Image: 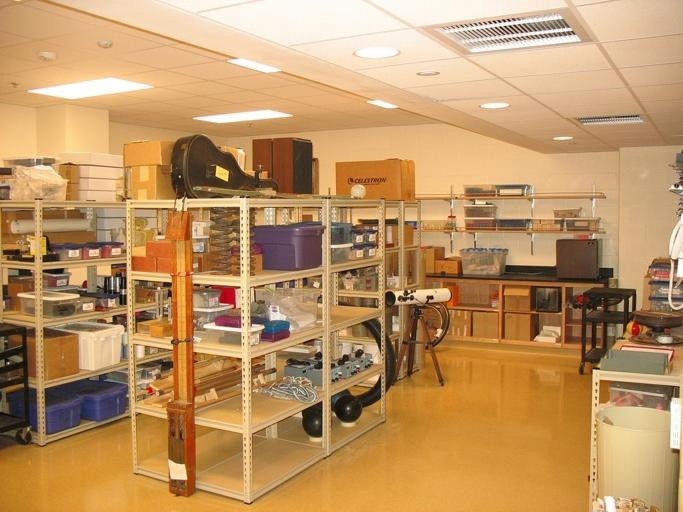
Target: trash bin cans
[595,406,679,512]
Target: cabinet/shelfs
[417,190,604,350]
[589,320,682,512]
[3,189,421,503]
[578,287,636,374]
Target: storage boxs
[251,137,312,194]
[124,140,177,199]
[335,158,415,202]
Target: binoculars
[385,288,452,314]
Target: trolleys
[0,323,31,444]
[579,287,636,374]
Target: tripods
[391,304,444,386]
